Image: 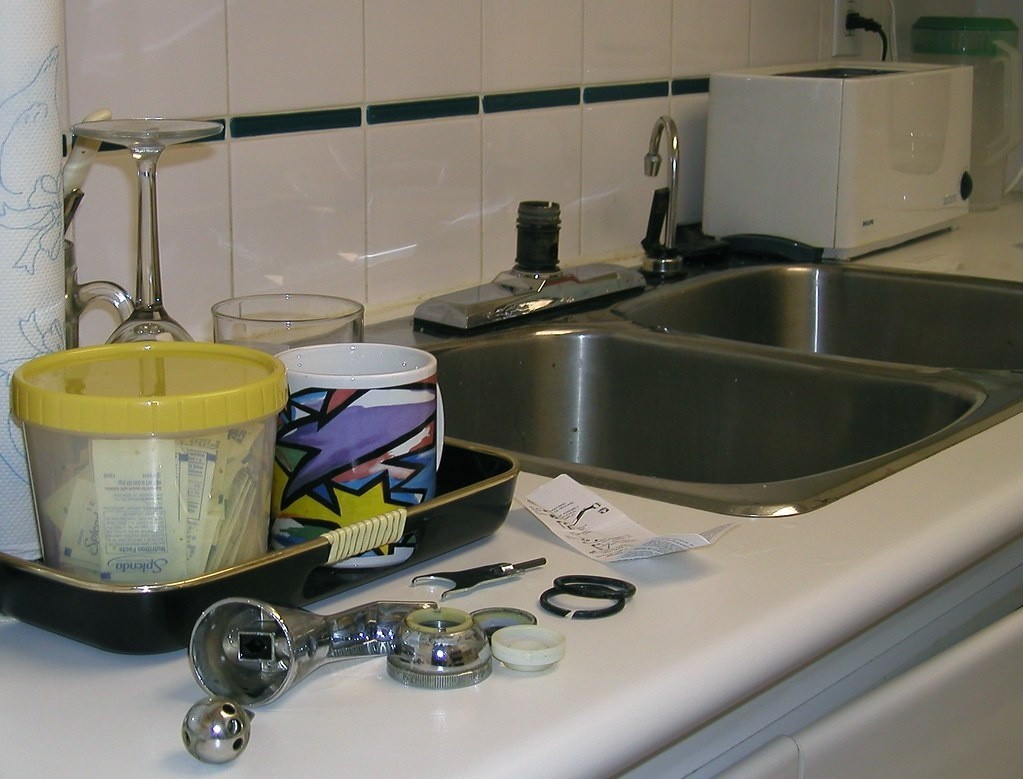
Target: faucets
[641,113,686,282]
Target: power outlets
[833,0,862,57]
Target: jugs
[911,13,1023,209]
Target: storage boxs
[11,341,289,587]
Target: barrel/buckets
[11,343,288,586]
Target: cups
[273,342,444,555]
[212,294,365,357]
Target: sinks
[614,261,1023,413]
[383,328,988,517]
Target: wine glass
[70,119,224,345]
